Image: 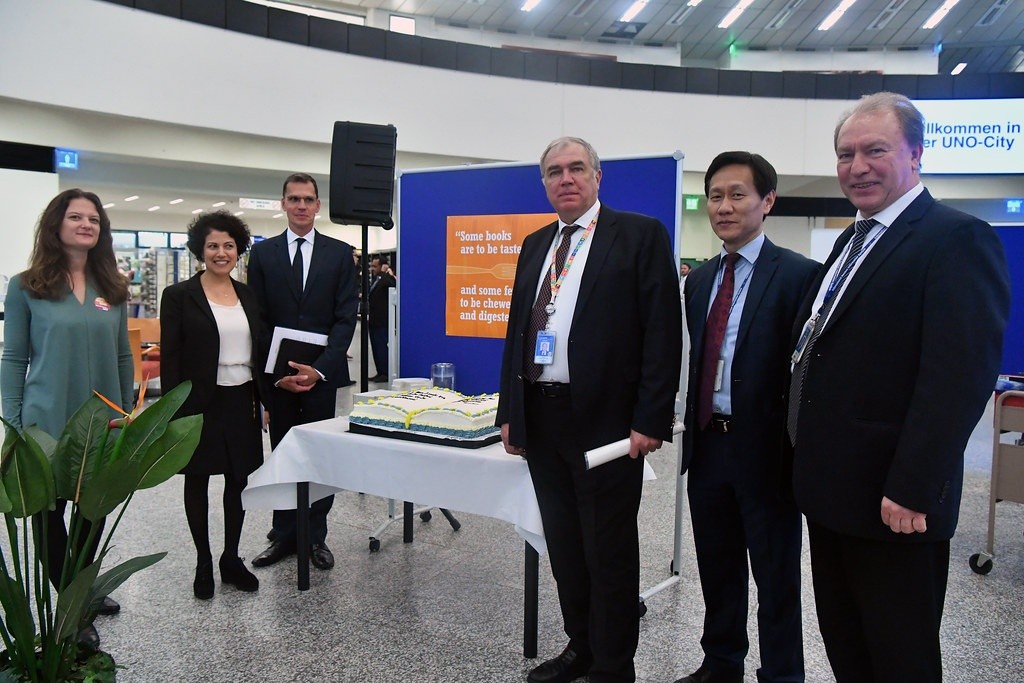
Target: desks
[241,415,684,675]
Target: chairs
[128,317,162,382]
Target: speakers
[330,120,397,230]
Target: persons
[247,173,358,571]
[680,262,691,294]
[336,245,397,388]
[495,136,682,683]
[790,91,1009,683]
[676,152,823,683]
[139,261,150,275]
[0,189,135,649]
[160,211,270,599]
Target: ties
[787,219,879,447]
[292,238,306,305]
[692,253,742,434]
[522,226,581,385]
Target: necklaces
[204,272,230,297]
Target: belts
[706,418,735,433]
[533,382,569,399]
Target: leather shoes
[251,540,298,568]
[527,640,591,683]
[78,623,101,650]
[311,542,335,570]
[97,594,120,614]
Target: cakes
[348,387,501,440]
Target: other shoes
[336,380,357,388]
[369,373,388,383]
[674,661,744,683]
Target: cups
[432,363,455,390]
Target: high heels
[193,551,259,599]
